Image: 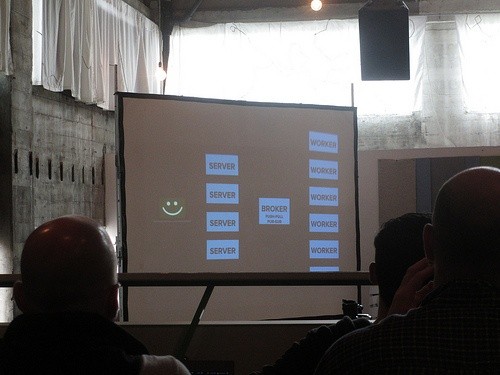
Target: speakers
[359,0,410,81]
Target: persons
[261,211,433,375]
[313,166,500,375]
[0,216,190,375]
